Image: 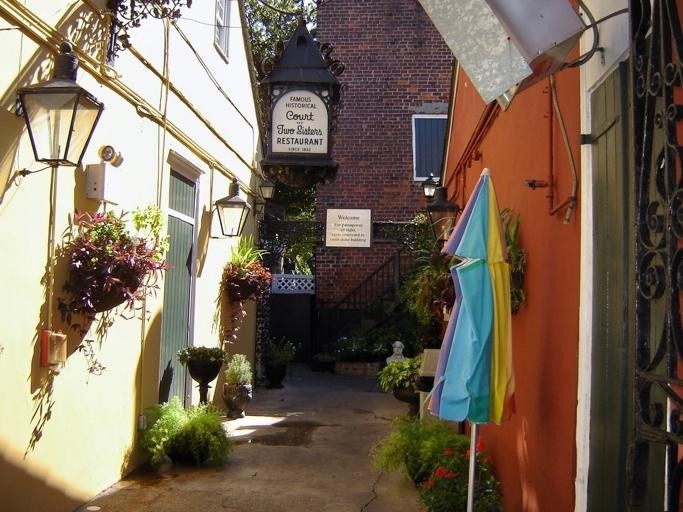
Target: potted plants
[59,204,170,373]
[222,233,273,346]
[177,344,228,403]
[140,395,234,470]
[223,353,253,418]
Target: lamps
[18,41,104,167]
[423,174,445,199]
[211,178,252,238]
[427,187,465,243]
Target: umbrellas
[427,168,527,511]
